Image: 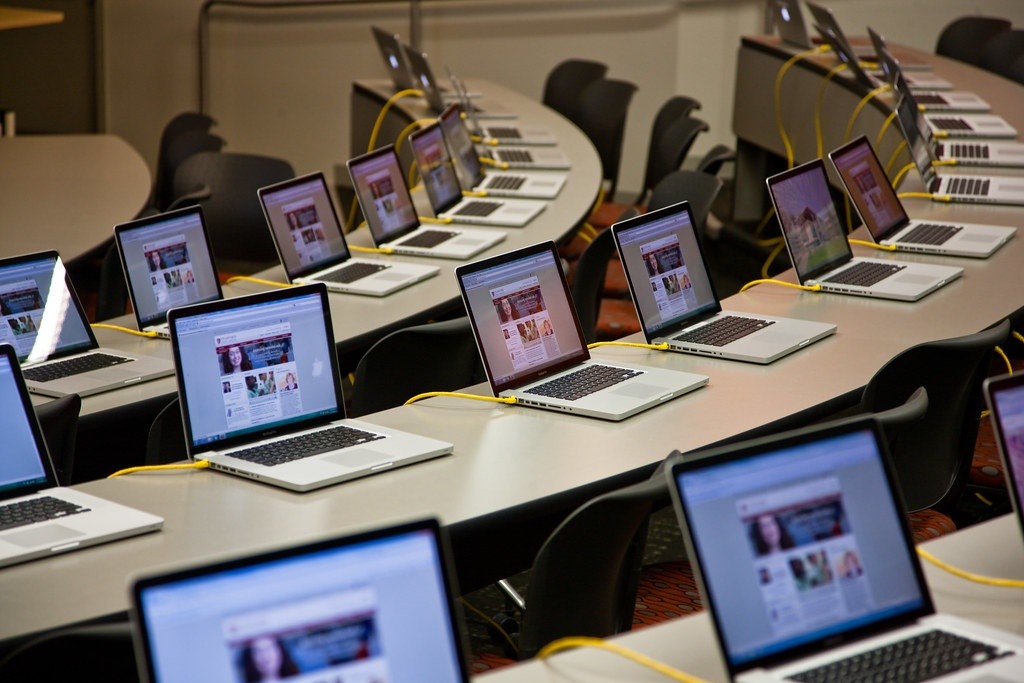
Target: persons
[149,251,195,286]
[645,253,693,293]
[371,183,381,199]
[497,296,554,340]
[286,211,323,245]
[222,346,298,392]
[234,634,296,681]
[746,512,863,585]
[7,314,37,333]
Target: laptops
[128,514,472,683]
[664,412,1024,683]
[0,0,1024,569]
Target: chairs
[0,16,1024,683]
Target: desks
[1,36,1024,644]
[26,81,602,430]
[0,135,153,265]
